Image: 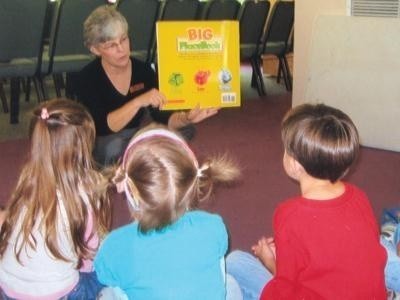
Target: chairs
[0,0,293,124]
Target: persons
[0,98,114,300]
[225,103,389,300]
[71,3,222,169]
[91,123,241,300]
